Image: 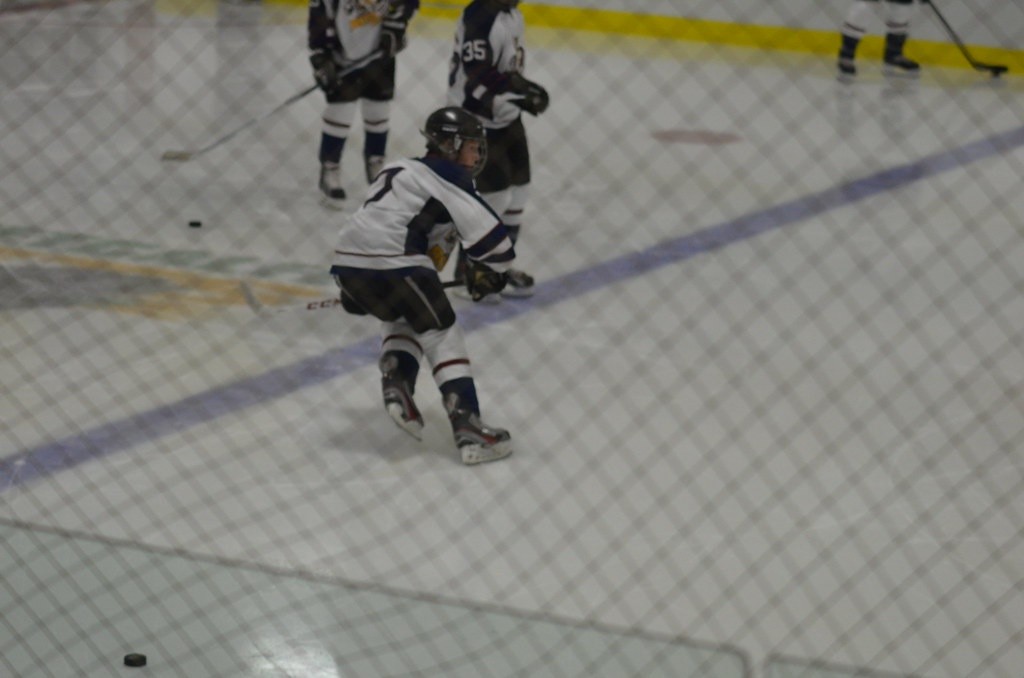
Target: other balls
[992,70,1000,77]
[123,653,146,667]
[188,220,202,228]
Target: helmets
[424,108,488,176]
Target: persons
[442,0,550,304]
[837,1,922,81]
[332,106,512,464]
[307,0,419,212]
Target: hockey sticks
[161,46,384,162]
[237,279,465,319]
[925,0,1009,72]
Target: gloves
[310,52,341,98]
[377,19,406,59]
[465,257,502,301]
[496,70,549,116]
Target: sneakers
[379,354,425,443]
[365,156,384,183]
[885,47,920,76]
[443,393,513,464]
[453,243,502,303]
[318,161,349,208]
[503,270,537,297]
[836,48,858,81]
